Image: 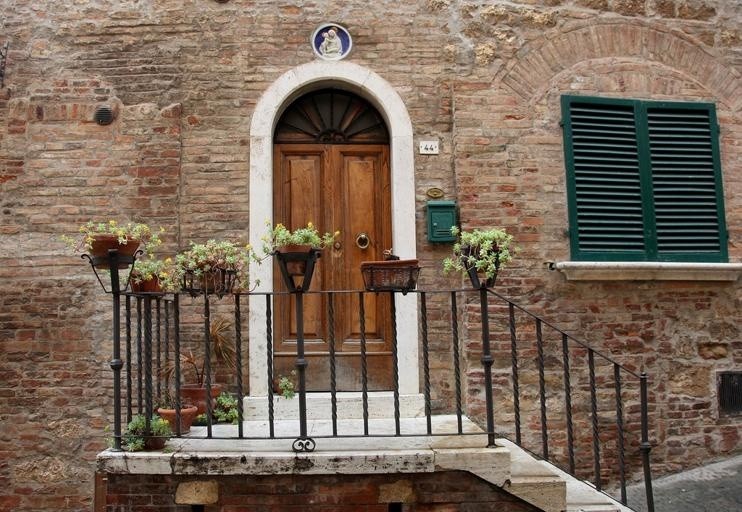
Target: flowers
[61,218,260,292]
[382,247,400,261]
[246,216,341,264]
[275,368,295,398]
[105,416,171,451]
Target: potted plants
[212,391,238,423]
[157,319,237,416]
[443,226,521,288]
[157,388,197,432]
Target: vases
[274,380,283,396]
[141,433,167,451]
[275,244,316,293]
[359,259,421,291]
